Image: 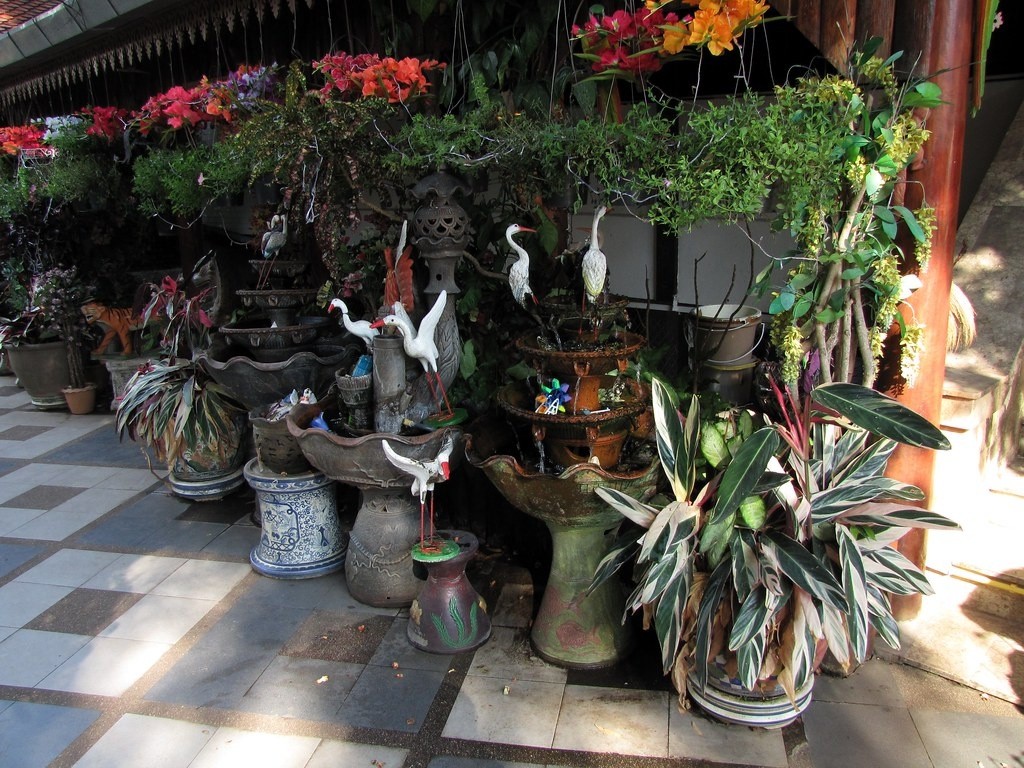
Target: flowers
[312,52,446,108]
[29,263,96,388]
[568,0,797,88]
[0,62,285,158]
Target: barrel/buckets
[690,304,766,365]
[693,356,762,407]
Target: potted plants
[586,370,963,729]
[0,310,96,410]
[113,274,247,502]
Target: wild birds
[328,288,458,423]
[506,223,539,310]
[575,202,615,308]
[254,214,291,289]
[381,435,456,554]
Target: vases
[61,383,96,414]
